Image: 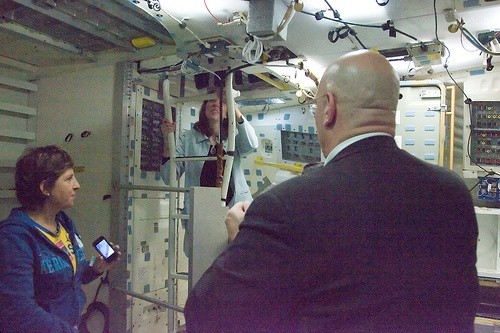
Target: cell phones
[92,236,118,263]
